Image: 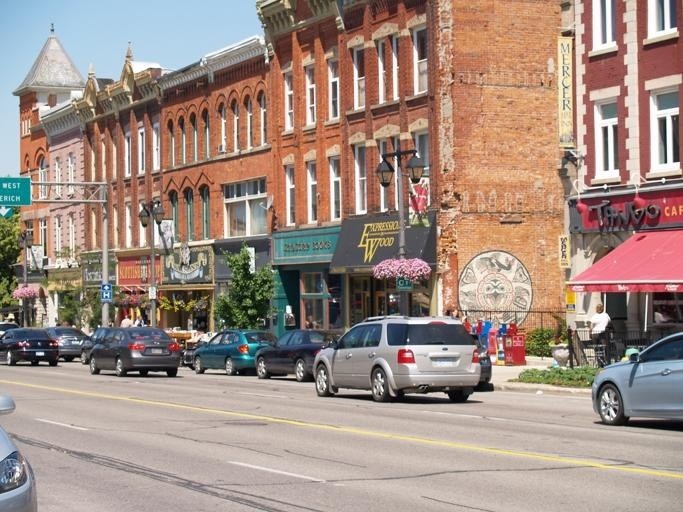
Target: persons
[590,304,611,367]
[120,314,148,328]
[63,319,77,328]
[654,305,668,324]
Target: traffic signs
[0,176,31,217]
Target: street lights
[375,147,425,317]
[18,231,34,328]
[138,200,165,326]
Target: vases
[550,344,569,367]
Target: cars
[0,396,37,511]
[592,333,683,425]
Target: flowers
[372,257,430,281]
[12,287,38,298]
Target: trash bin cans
[605,341,627,365]
